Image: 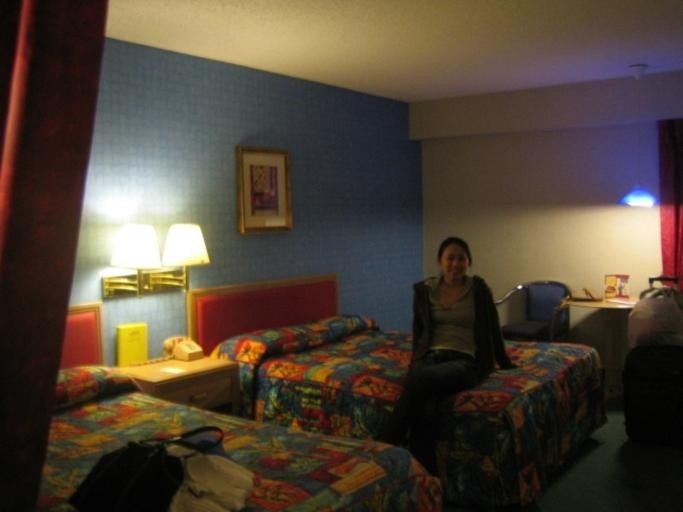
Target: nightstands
[115,356,243,417]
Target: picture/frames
[236,148,294,234]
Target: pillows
[53,366,142,411]
[220,313,377,367]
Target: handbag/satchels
[627,287,683,347]
[68,440,183,512]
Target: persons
[375,237,518,477]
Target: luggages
[622,277,683,443]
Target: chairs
[494,281,571,342]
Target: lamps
[100,223,212,302]
[617,64,659,208]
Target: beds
[187,274,605,506]
[40,302,443,512]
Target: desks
[560,291,640,408]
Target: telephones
[163,335,204,361]
